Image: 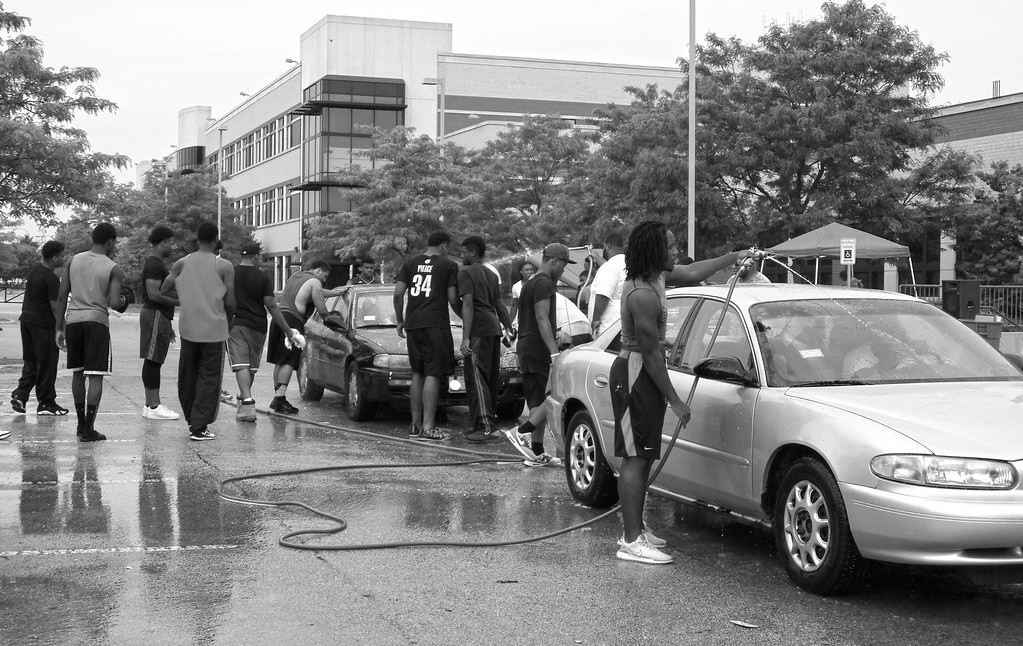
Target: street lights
[217,125,228,240]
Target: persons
[608,222,758,564]
[11,221,770,469]
[839,270,864,288]
[843,316,939,379]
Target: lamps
[286,59,298,66]
[240,92,251,98]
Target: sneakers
[523,452,562,468]
[37,399,69,415]
[616,520,668,548]
[505,425,538,461]
[616,530,674,563]
[142,403,179,420]
[10,394,26,413]
[188,425,216,440]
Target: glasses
[735,264,742,266]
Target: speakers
[941,280,980,321]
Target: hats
[239,242,261,255]
[542,242,578,265]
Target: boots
[80,404,107,442]
[75,402,86,436]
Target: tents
[761,222,919,299]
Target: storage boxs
[958,319,1003,352]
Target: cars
[542,281,1023,599]
[296,282,526,420]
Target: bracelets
[551,353,560,357]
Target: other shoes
[462,428,504,443]
[0,430,12,440]
[235,393,257,422]
[269,395,299,413]
[218,390,233,401]
[408,423,451,442]
